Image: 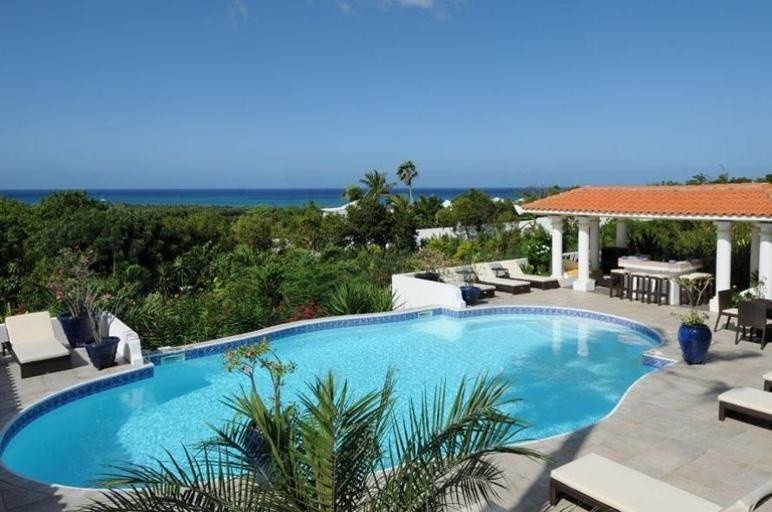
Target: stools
[630,270,650,305]
[608,269,631,300]
[647,274,671,307]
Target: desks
[740,282,771,314]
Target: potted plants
[80,276,140,370]
[673,275,714,370]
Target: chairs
[761,369,772,393]
[0,309,75,379]
[716,386,772,427]
[714,288,741,333]
[434,259,563,306]
[734,298,771,350]
[544,448,772,510]
